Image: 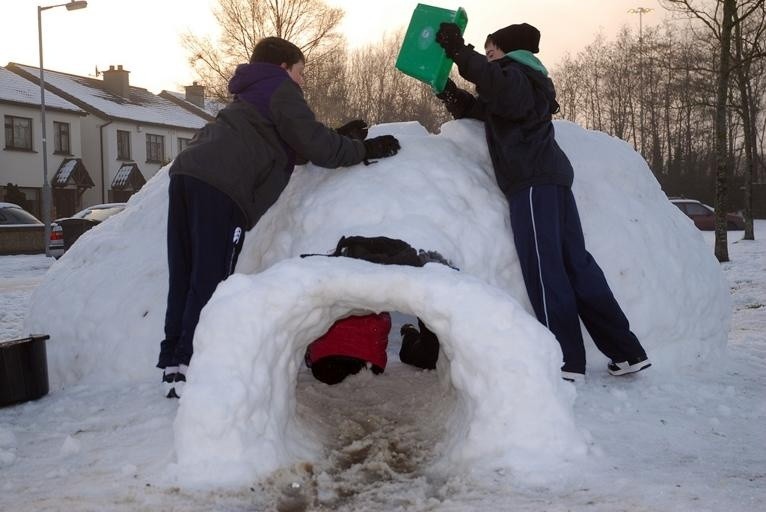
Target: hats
[492,23,540,54]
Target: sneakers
[607,355,652,376]
[161,372,186,398]
[561,370,585,382]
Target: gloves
[335,120,368,140]
[437,77,470,115]
[363,135,400,159]
[436,23,464,58]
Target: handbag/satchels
[343,236,422,267]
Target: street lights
[35,0,92,258]
[627,6,657,163]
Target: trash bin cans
[58,219,102,254]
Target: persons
[155,35,404,402]
[431,18,657,387]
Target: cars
[0,203,43,225]
[46,201,127,264]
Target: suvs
[667,193,745,231]
